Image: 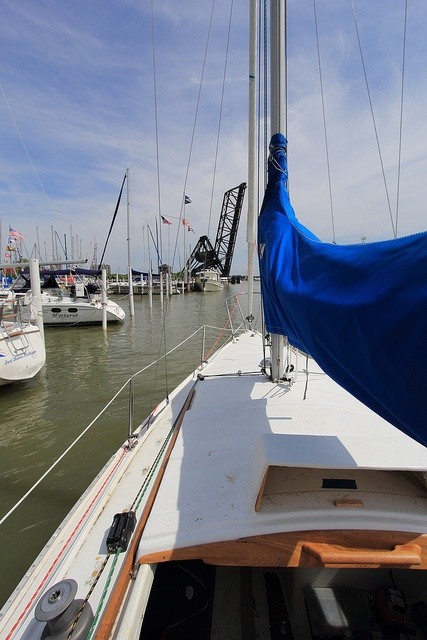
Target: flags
[185,194,192,205]
[8,226,25,239]
[180,218,196,235]
[161,215,172,225]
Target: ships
[195,269,224,292]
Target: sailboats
[0,225,103,313]
[109,167,181,294]
[0,0,427,640]
[16,233,126,323]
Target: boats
[0,299,46,386]
[221,277,228,284]
